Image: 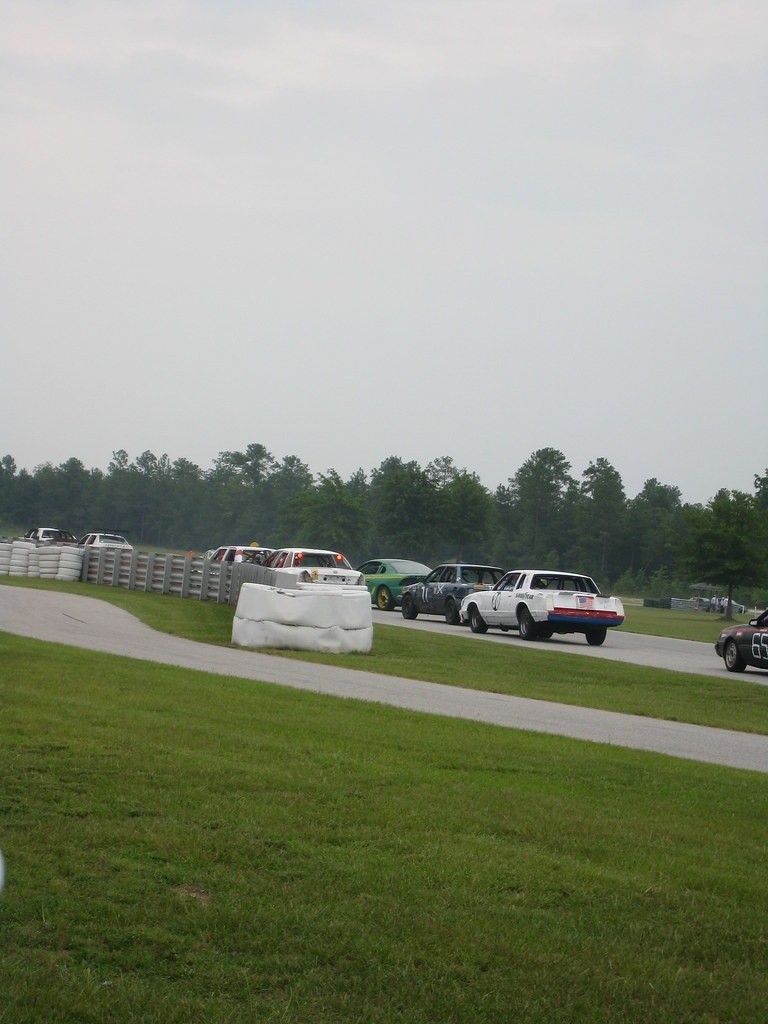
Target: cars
[725,598,747,614]
[400,563,515,626]
[191,545,276,575]
[77,532,134,552]
[715,607,768,672]
[353,558,439,612]
[458,569,625,646]
[262,547,366,587]
[17,527,78,542]
